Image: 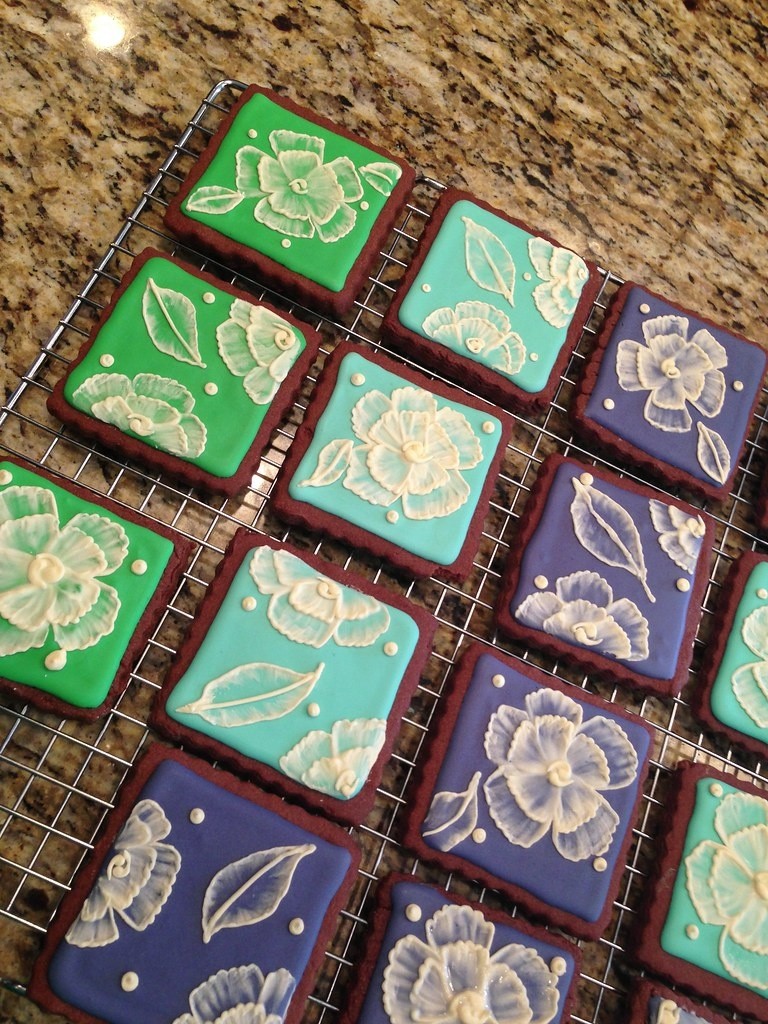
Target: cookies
[0,79,768,1024]
